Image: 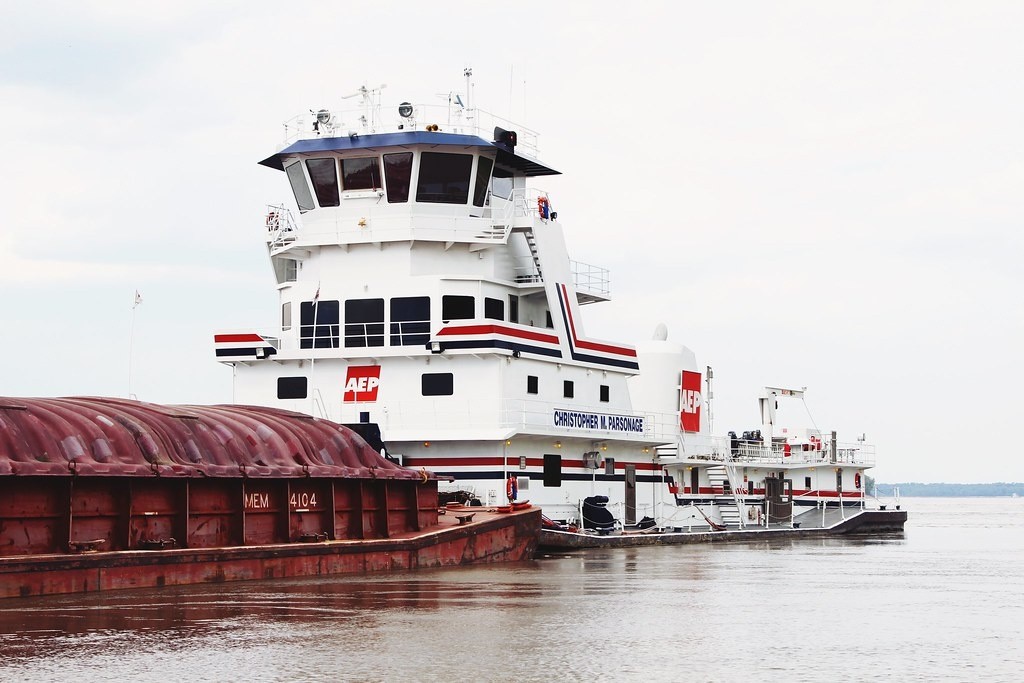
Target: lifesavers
[507,477,518,500]
[855,473,861,488]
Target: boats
[214,67,907,547]
